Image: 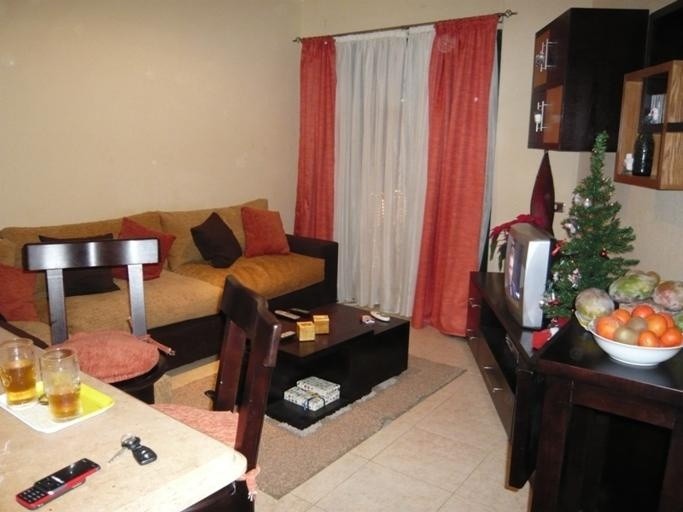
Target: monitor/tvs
[503,222,567,330]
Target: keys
[109,433,157,465]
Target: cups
[0,338,39,410]
[40,347,81,423]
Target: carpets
[159,339,467,499]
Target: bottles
[631,124,655,178]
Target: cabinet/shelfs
[527,88,621,152]
[532,7,647,87]
[614,60,683,193]
[460,272,562,493]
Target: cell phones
[16,459,100,510]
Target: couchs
[1,199,339,372]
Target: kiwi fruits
[627,316,647,331]
[614,325,639,346]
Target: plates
[0,381,115,434]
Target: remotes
[290,307,309,314]
[274,310,301,321]
[371,310,390,321]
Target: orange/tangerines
[660,325,683,347]
[610,308,632,326]
[631,304,655,319]
[657,311,674,328]
[644,314,667,337]
[596,315,622,340]
[637,330,659,348]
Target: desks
[1,330,248,512]
[532,295,683,512]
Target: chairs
[23,236,164,423]
[142,275,282,511]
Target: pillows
[37,233,120,300]
[113,217,177,281]
[189,212,242,268]
[0,262,37,323]
[241,206,291,259]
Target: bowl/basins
[588,320,683,366]
